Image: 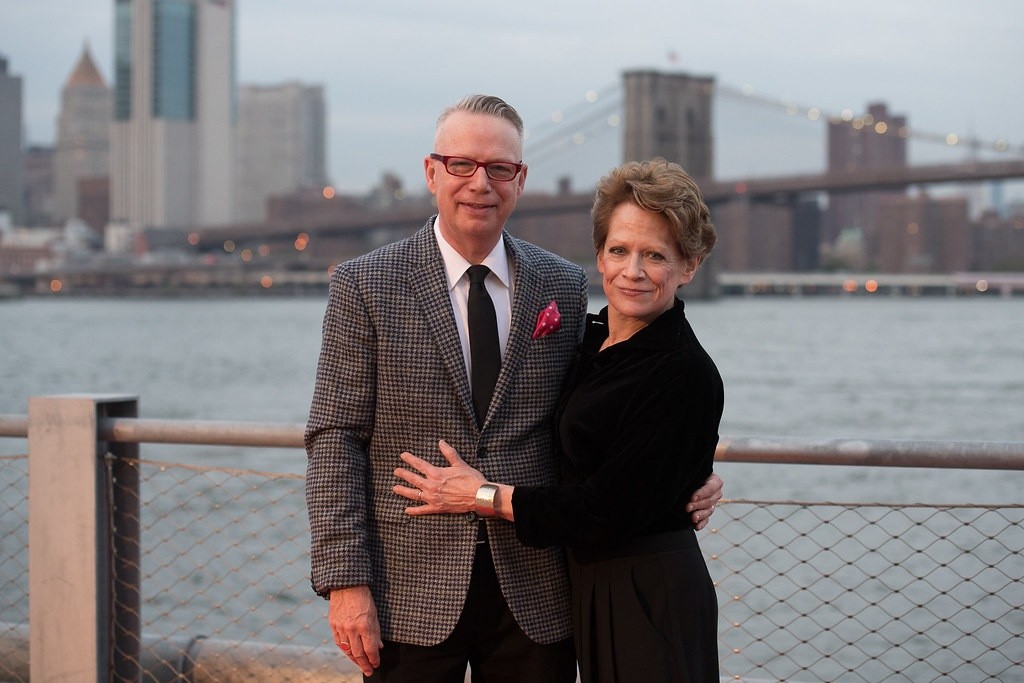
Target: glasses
[428,150,520,182]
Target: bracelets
[475,485,498,517]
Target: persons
[299,93,588,682]
[392,160,725,682]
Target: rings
[418,490,422,501]
[711,505,715,512]
[340,642,350,651]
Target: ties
[465,265,501,430]
